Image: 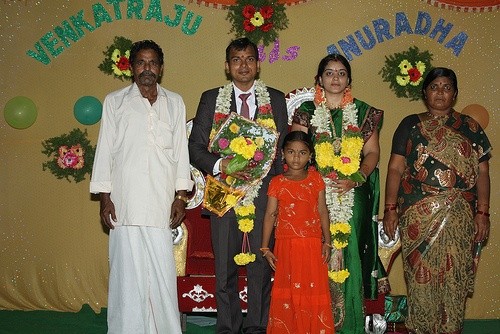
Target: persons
[262,131,335,334]
[189,38,288,334]
[383,67,494,334]
[90,40,187,334]
[292,54,391,334]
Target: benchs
[171,118,403,334]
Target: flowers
[212,117,277,205]
[41,128,97,183]
[377,45,435,101]
[96,35,134,83]
[224,0,289,47]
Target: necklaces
[209,79,277,265]
[311,84,364,248]
[427,112,445,118]
[325,98,345,153]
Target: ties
[239,93,250,120]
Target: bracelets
[263,250,270,257]
[477,211,491,217]
[355,170,367,188]
[384,207,396,214]
[476,204,490,208]
[385,204,397,206]
[324,241,334,249]
[174,195,187,203]
[260,248,270,252]
